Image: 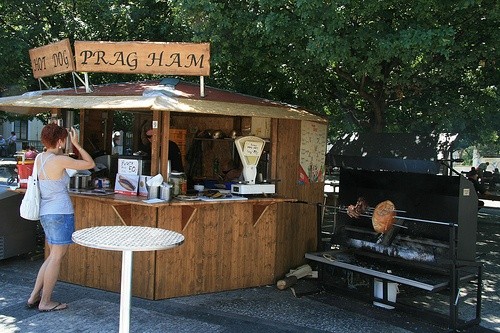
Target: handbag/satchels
[20,153,42,221]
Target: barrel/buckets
[16,164,33,190]
[373,277,399,310]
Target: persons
[6,131,17,143]
[0,135,6,144]
[25,145,47,159]
[23,123,95,313]
[143,124,183,177]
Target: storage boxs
[169,129,187,169]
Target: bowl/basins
[159,184,174,201]
[146,185,160,200]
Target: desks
[71,225,184,333]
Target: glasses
[145,134,152,139]
[58,126,62,130]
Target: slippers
[38,302,68,312]
[25,296,41,308]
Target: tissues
[146,173,163,199]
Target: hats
[0,135,4,138]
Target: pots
[70,174,93,190]
